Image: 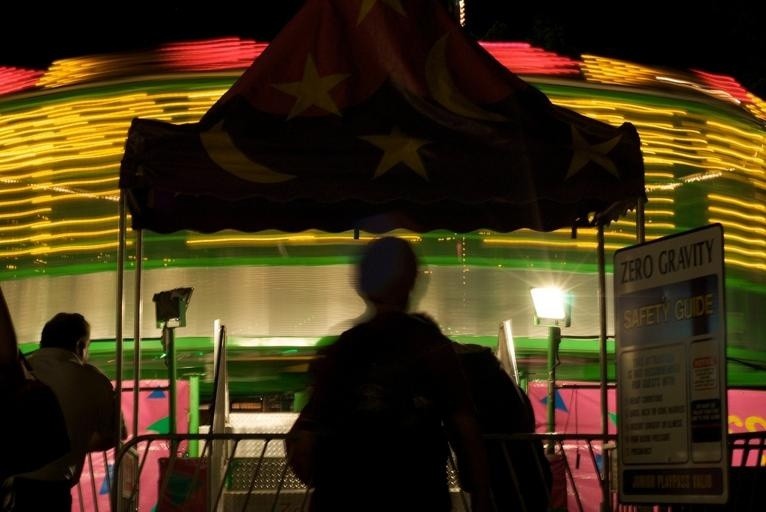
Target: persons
[0,313,127,512]
[0,289,71,512]
[306,237,490,512]
[452,345,552,512]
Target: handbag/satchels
[17,350,72,475]
